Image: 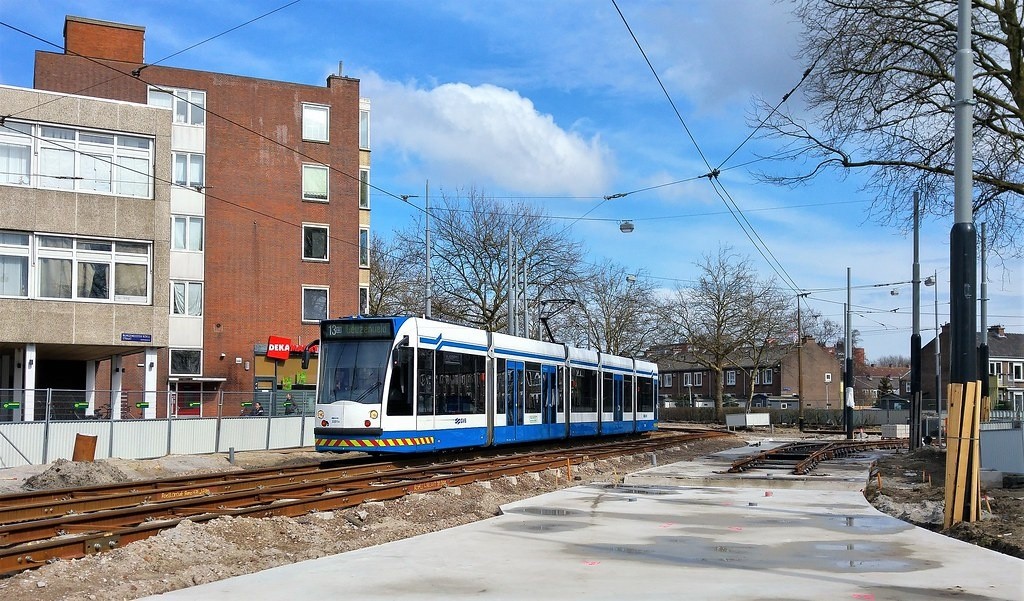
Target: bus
[300,314,661,458]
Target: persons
[247,401,264,416]
[281,392,298,415]
